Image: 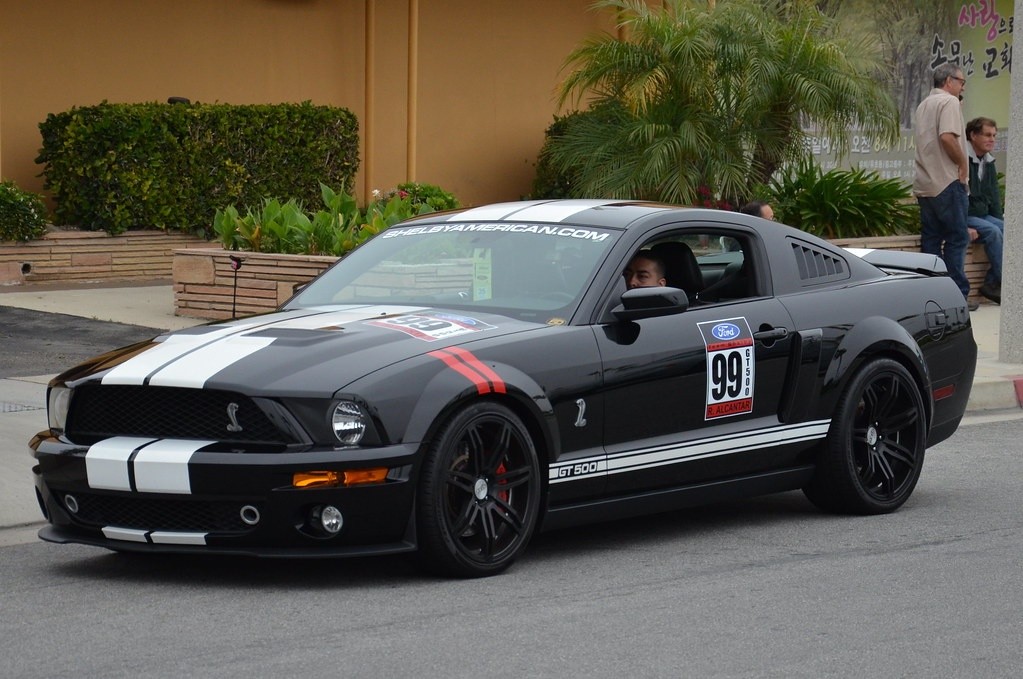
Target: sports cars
[29,198,980,579]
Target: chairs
[651,241,718,308]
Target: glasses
[951,76,966,86]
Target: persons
[623,250,669,292]
[955,118,1007,308]
[727,203,782,252]
[913,63,980,312]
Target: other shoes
[979,285,1001,304]
[966,301,979,311]
